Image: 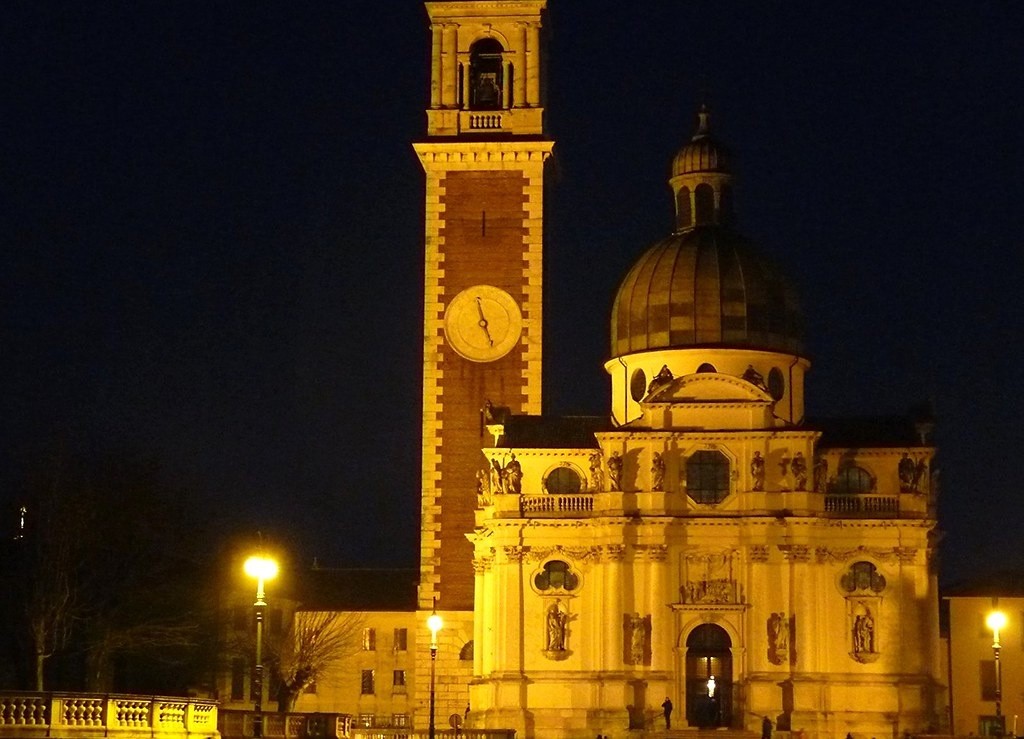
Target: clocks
[443,285,523,363]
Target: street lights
[242,544,279,738]
[983,610,1007,738]
[426,595,444,738]
